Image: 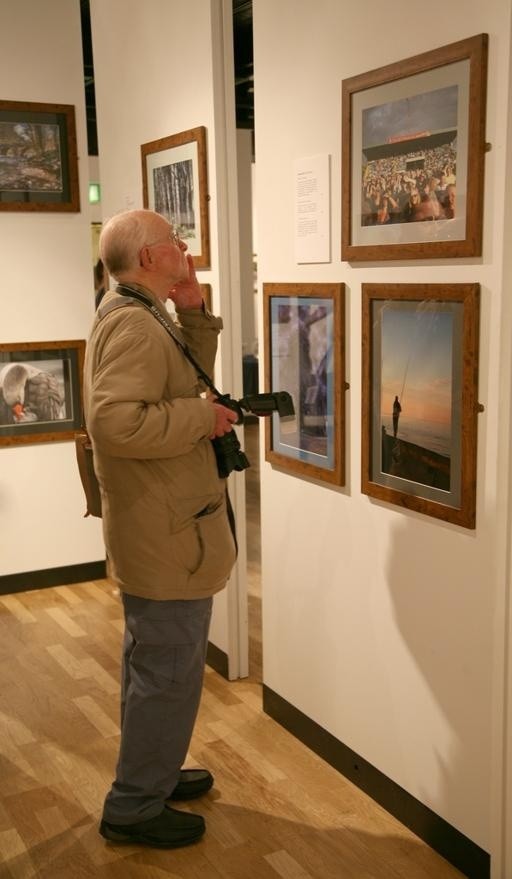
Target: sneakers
[166,768,215,802]
[97,803,208,849]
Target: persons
[392,395,401,437]
[361,142,456,226]
[12,401,37,423]
[84,209,237,851]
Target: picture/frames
[0,338,87,449]
[0,97,82,214]
[261,279,347,486]
[359,282,480,533]
[341,32,489,259]
[141,124,211,270]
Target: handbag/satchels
[73,295,144,519]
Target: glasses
[410,194,417,198]
[148,229,180,247]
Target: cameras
[210,390,297,479]
